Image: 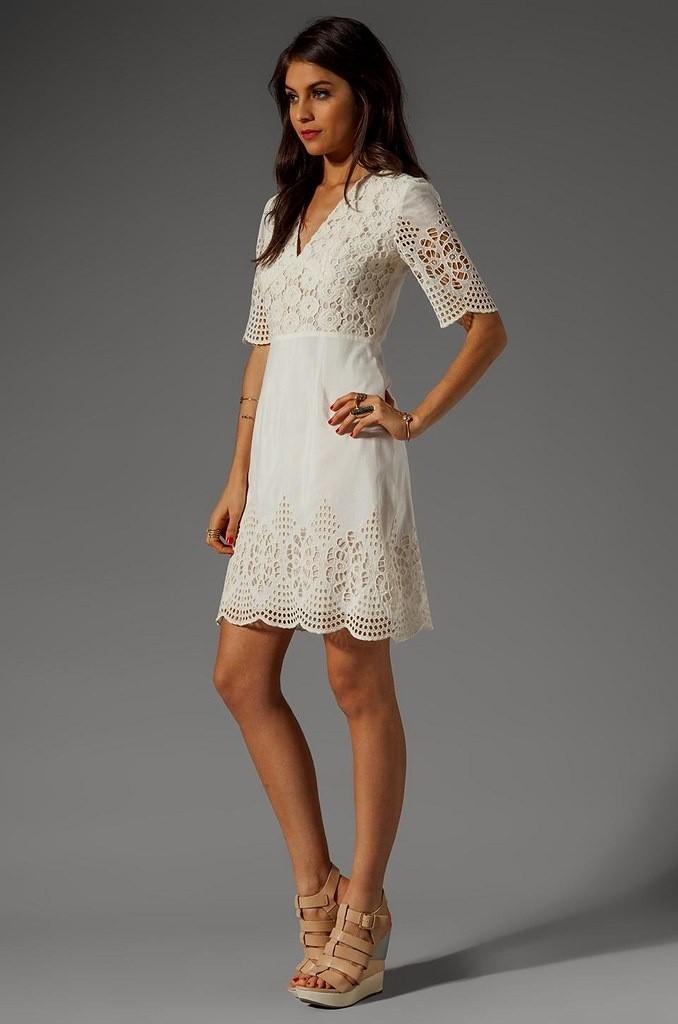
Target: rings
[206,528,221,539]
[351,390,374,415]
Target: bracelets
[239,393,258,420]
[402,411,412,443]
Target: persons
[201,17,509,1008]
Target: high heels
[297,889,392,1008]
[287,862,340,999]
